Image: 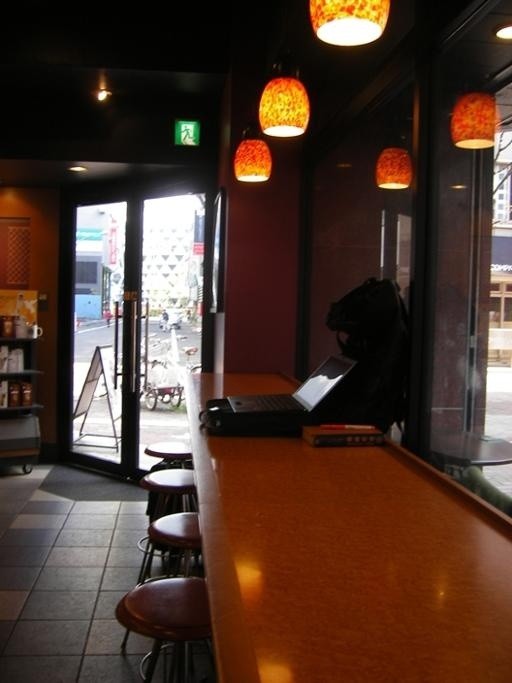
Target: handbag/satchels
[198,398,303,438]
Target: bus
[165,296,197,320]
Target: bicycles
[152,336,202,376]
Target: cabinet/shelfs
[2,333,43,466]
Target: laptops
[226,351,358,414]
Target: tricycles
[140,360,184,410]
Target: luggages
[145,457,199,555]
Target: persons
[161,309,169,332]
[105,309,113,325]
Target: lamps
[450,87,502,150]
[310,1,391,48]
[375,144,413,191]
[259,52,310,139]
[233,119,272,187]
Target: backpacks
[325,277,414,434]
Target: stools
[116,441,213,683]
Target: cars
[159,312,182,329]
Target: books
[304,423,385,447]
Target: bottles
[0,315,44,410]
